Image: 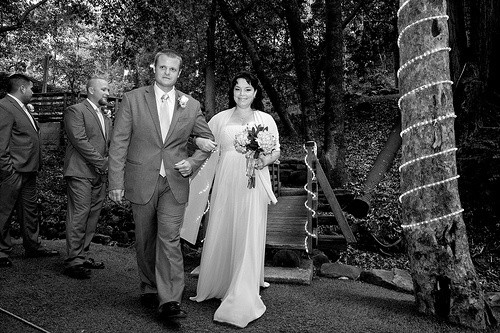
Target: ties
[23,106,35,128]
[159,94,169,178]
[95,108,105,137]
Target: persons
[107,48,216,320]
[179,70,281,328]
[63,78,112,276]
[0,74,60,268]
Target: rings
[257,164,259,168]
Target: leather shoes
[0,257,12,268]
[82,259,104,268]
[29,249,58,257]
[158,301,187,317]
[64,265,91,275]
[140,291,158,309]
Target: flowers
[104,109,111,118]
[26,103,35,112]
[177,95,189,108]
[233,122,281,188]
